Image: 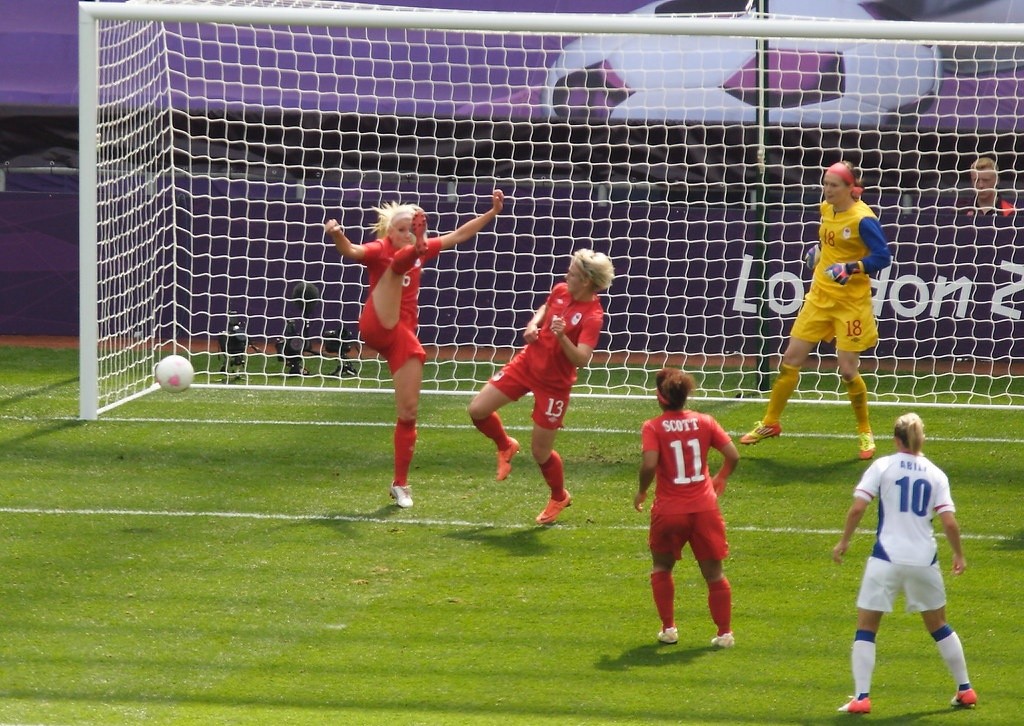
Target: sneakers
[838,696,871,713]
[496,437,520,480]
[951,689,976,707]
[858,431,875,460]
[711,633,734,647]
[389,480,413,507]
[658,627,678,643]
[535,489,571,524]
[411,211,429,255]
[742,423,781,445]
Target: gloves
[826,262,853,285]
[803,244,820,270]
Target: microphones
[292,282,320,310]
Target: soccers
[157,354,196,391]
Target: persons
[324,189,504,508]
[635,368,739,647]
[467,249,615,525]
[833,413,978,713]
[962,157,1022,216]
[739,160,891,460]
